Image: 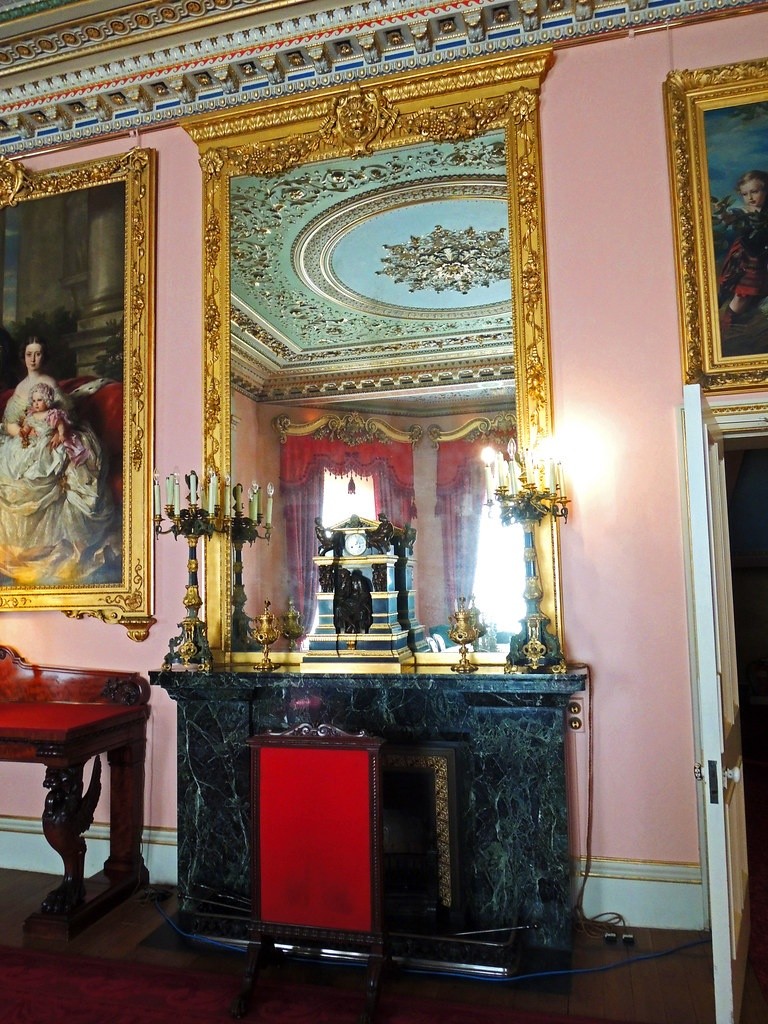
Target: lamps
[151,465,231,671]
[230,481,274,652]
[482,437,571,675]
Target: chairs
[432,633,446,651]
[425,636,438,653]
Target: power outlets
[566,698,584,732]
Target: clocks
[303,513,411,663]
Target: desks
[0,645,150,943]
[441,643,511,653]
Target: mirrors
[181,46,564,668]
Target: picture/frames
[0,146,157,644]
[662,55,768,396]
